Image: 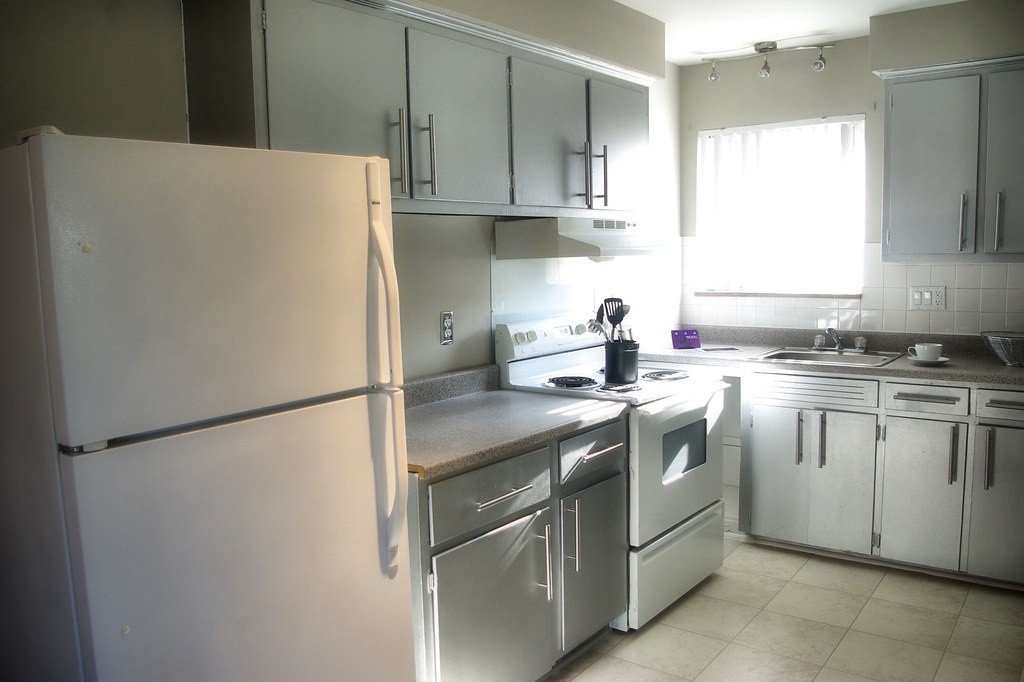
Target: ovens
[603,380,723,633]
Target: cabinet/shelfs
[738,370,880,566]
[408,417,628,682]
[880,56,1024,263]
[877,376,1024,591]
[183,1,652,216]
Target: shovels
[604,298,624,342]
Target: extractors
[490,216,659,263]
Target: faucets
[826,326,843,349]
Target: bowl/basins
[980,329,1024,365]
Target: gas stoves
[494,314,724,406]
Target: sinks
[762,346,895,366]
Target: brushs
[586,319,613,343]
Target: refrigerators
[0,124,418,682]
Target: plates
[908,356,950,366]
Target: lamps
[708,61,720,82]
[812,47,825,72]
[757,53,771,79]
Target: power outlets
[909,286,945,310]
[439,311,454,345]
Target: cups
[603,340,639,384]
[908,343,943,360]
